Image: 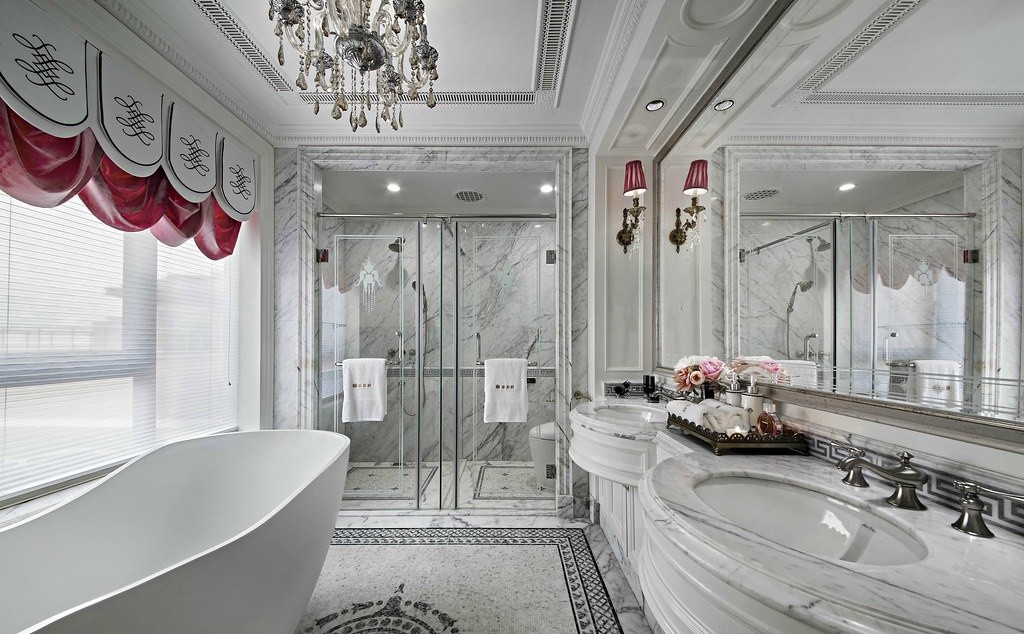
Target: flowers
[732,355,790,386]
[672,353,736,402]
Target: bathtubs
[0,427,352,634]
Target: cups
[725,389,744,407]
[741,392,765,427]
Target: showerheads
[787,280,814,312]
[805,233,833,253]
[411,280,428,310]
[388,236,406,253]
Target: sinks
[691,469,929,565]
[593,403,670,423]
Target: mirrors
[651,0,1024,458]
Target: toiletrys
[724,372,783,438]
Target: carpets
[294,526,625,634]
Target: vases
[700,381,716,400]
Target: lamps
[267,0,440,134]
[614,159,647,255]
[669,159,709,254]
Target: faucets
[651,390,686,406]
[836,450,930,512]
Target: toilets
[528,419,571,491]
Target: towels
[905,359,965,414]
[483,358,529,424]
[765,359,819,391]
[342,358,389,424]
[666,398,751,437]
